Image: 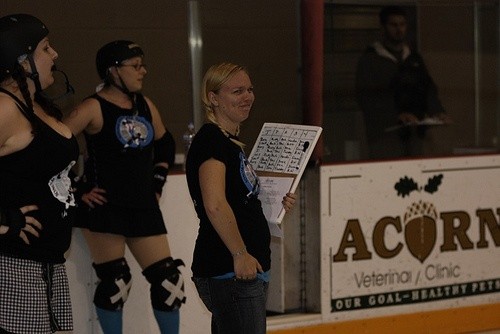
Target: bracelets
[231,246,246,257]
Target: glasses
[120,63,148,71]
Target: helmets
[0,13,49,63]
[96,40,144,80]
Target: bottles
[183,121,195,171]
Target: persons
[63,39,186,334]
[185,64,298,334]
[0,14,80,334]
[359,5,447,157]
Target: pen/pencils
[296,141,309,170]
